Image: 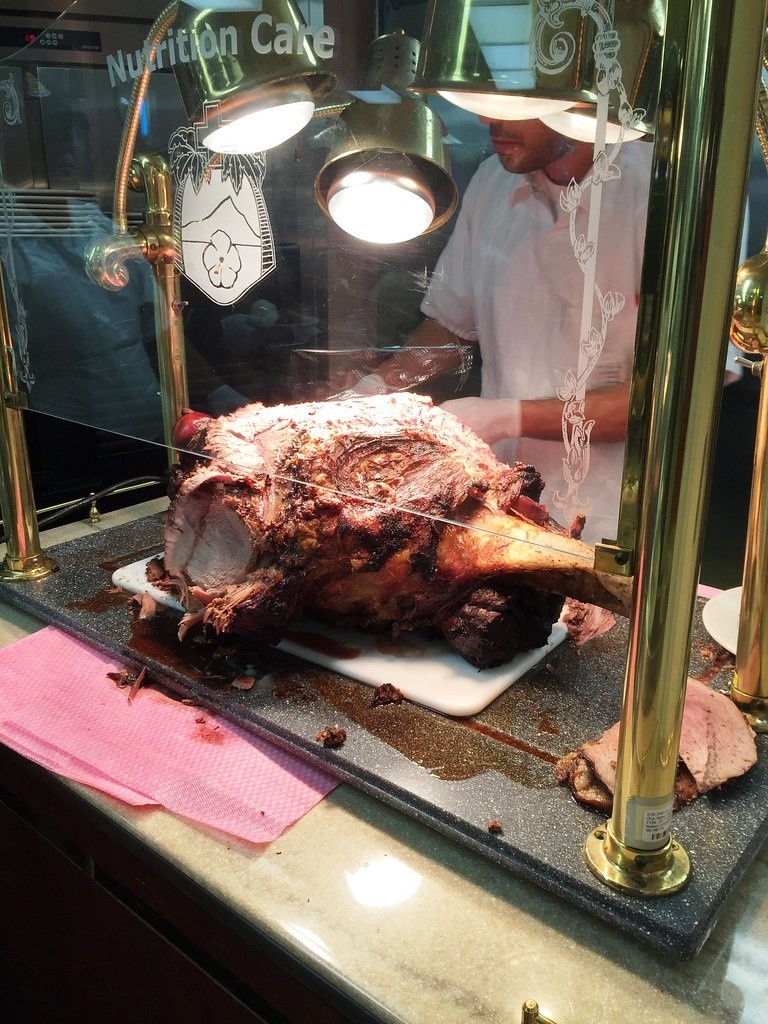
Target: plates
[702,586,743,655]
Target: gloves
[436,397,522,445]
[351,373,388,395]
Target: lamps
[203,27,458,245]
[405,0,768,178]
[82,0,338,292]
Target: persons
[0,86,253,538]
[334,114,751,552]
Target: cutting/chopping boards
[110,550,568,717]
